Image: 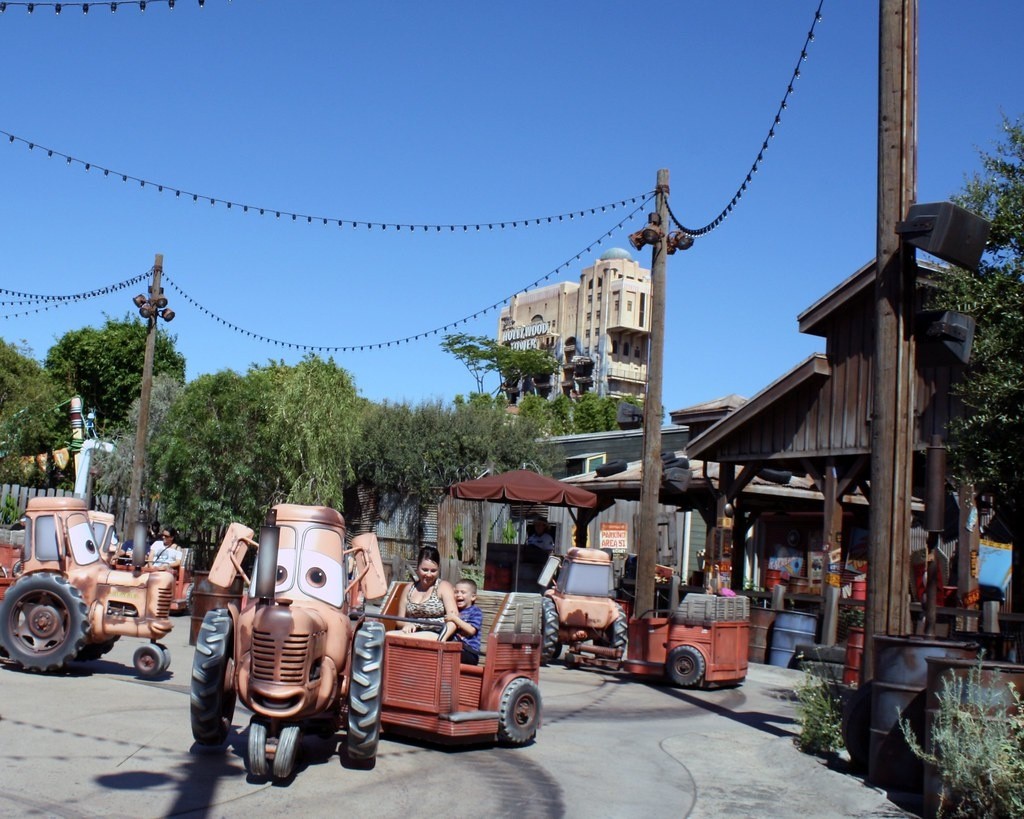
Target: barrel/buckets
[768,610,817,668]
[850,579,866,617]
[692,569,703,586]
[788,575,808,594]
[748,605,777,665]
[765,568,781,592]
[189,571,244,646]
[923,656,1024,819]
[864,633,979,795]
[840,625,864,774]
[617,598,631,626]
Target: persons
[386,546,460,642]
[111,526,153,568]
[527,516,554,555]
[444,578,483,665]
[145,527,182,575]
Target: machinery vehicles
[188,504,546,780]
[0,497,194,678]
[537,546,752,688]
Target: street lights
[625,168,694,619]
[127,253,175,530]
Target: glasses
[161,534,171,538]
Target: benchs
[366,581,542,711]
[642,592,750,663]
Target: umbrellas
[451,462,598,591]
